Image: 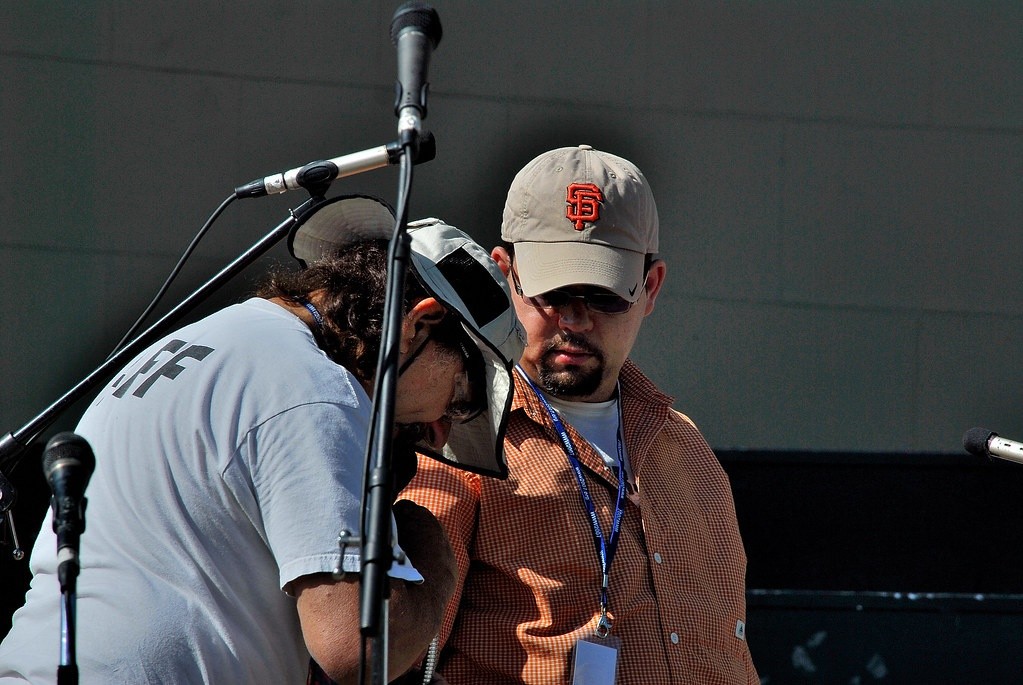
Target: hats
[286,194,528,482]
[501,144,659,303]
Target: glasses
[510,258,650,316]
[442,318,487,424]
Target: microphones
[963,427,1023,464]
[43,432,95,593]
[235,130,437,198]
[390,0,442,147]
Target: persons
[1,215,526,685]
[397,143,767,685]
